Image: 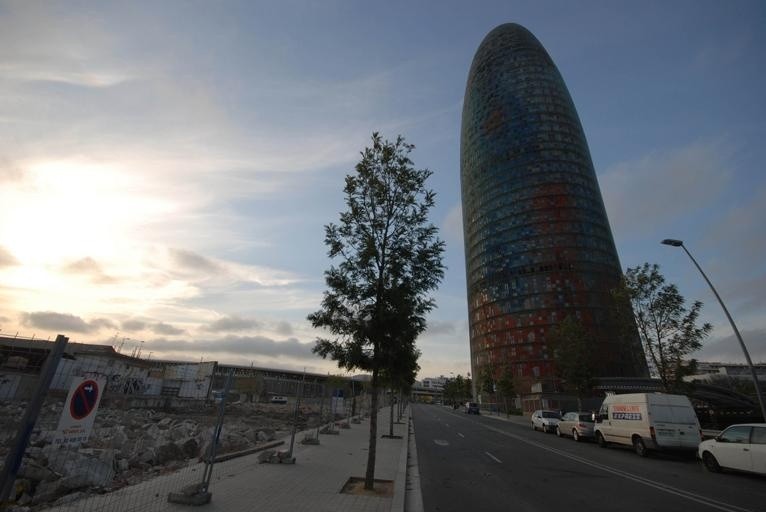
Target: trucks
[593,391,704,459]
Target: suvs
[465,402,480,414]
[532,409,562,432]
[556,411,595,441]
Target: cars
[697,423,766,477]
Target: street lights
[659,237,766,420]
[450,371,456,383]
